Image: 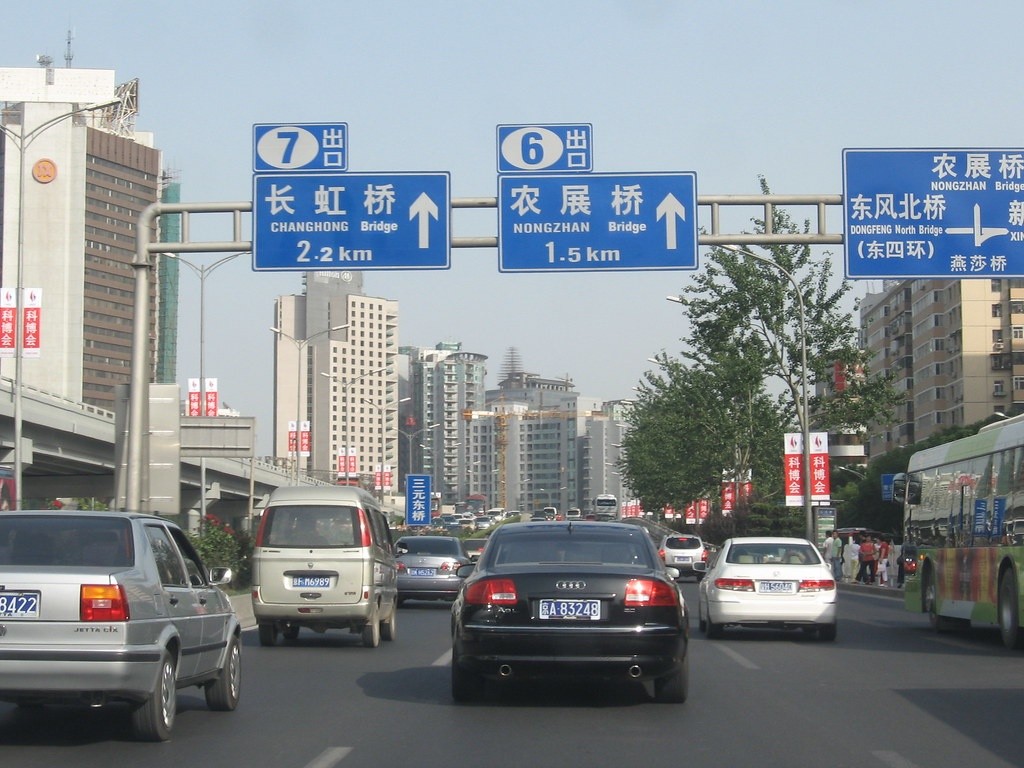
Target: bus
[891,412,1024,650]
[592,494,618,522]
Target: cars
[462,538,489,564]
[692,538,838,641]
[0,512,244,742]
[431,507,582,532]
[656,533,709,581]
[393,535,474,609]
[450,519,690,703]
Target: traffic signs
[841,147,1024,279]
[497,170,699,273]
[251,170,452,272]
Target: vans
[251,485,409,649]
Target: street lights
[13,95,124,510]
[712,244,812,538]
[269,325,566,506]
[161,252,252,537]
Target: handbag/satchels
[877,561,885,572]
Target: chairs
[601,544,634,564]
[790,555,802,563]
[505,542,540,563]
[736,554,754,564]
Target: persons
[821,531,906,589]
[545,513,563,521]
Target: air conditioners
[995,342,1005,350]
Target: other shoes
[863,582,872,585]
[878,583,888,587]
[897,585,902,588]
